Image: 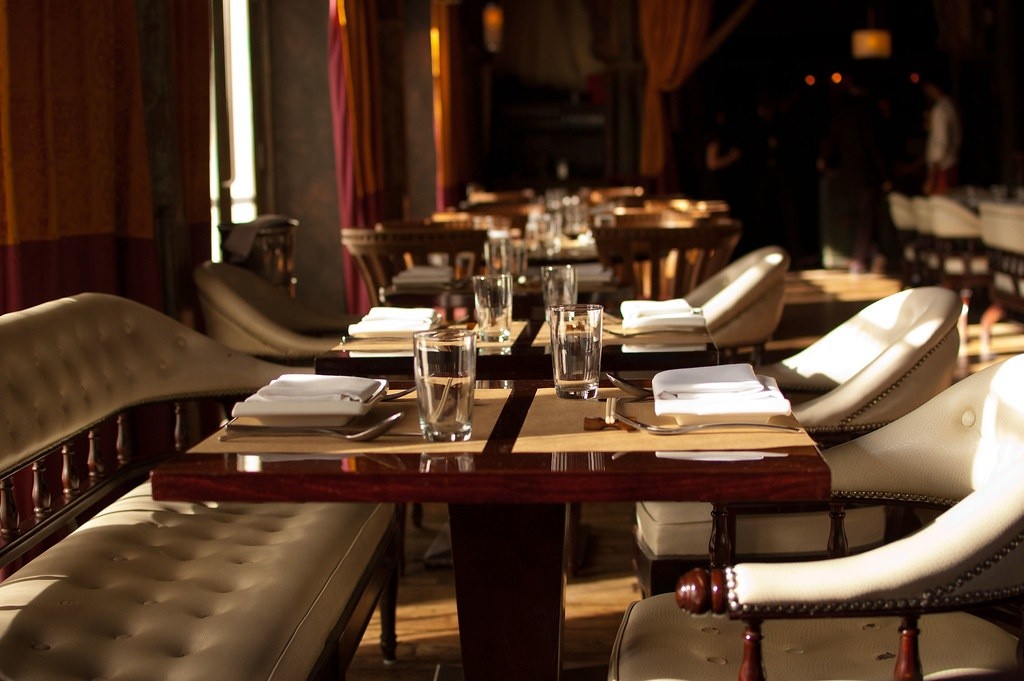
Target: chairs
[192,261,358,367]
[881,193,1024,313]
[340,184,794,349]
[605,285,1024,681]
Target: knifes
[598,396,654,403]
[225,425,422,436]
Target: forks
[613,411,799,435]
[382,372,434,401]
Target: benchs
[0,291,406,681]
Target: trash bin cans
[219,215,300,299]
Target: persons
[699,62,1001,268]
[921,72,965,195]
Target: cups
[472,274,513,342]
[414,328,476,441]
[487,235,512,273]
[541,266,577,321]
[549,305,603,399]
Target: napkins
[652,362,792,419]
[620,298,705,337]
[392,266,454,284]
[231,373,389,416]
[347,306,444,339]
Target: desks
[316,310,716,382]
[390,250,615,293]
[149,319,834,681]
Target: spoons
[606,372,652,397]
[602,328,705,338]
[218,412,404,442]
